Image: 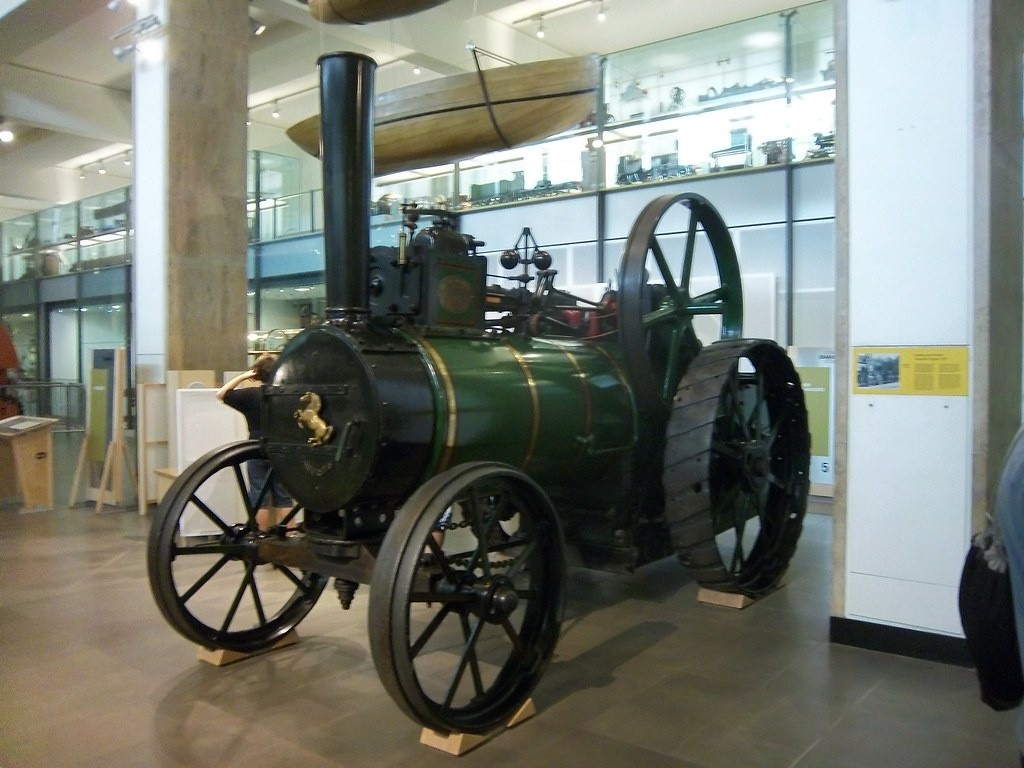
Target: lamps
[273,102,279,119]
[108,1,128,14]
[112,44,135,59]
[535,16,545,38]
[597,0,607,20]
[75,149,133,180]
[247,16,266,36]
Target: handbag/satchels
[959,533,1022,711]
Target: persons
[216,353,294,538]
[993,430,1024,768]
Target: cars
[146,51,812,734]
